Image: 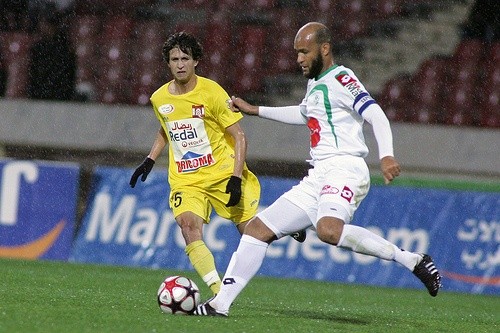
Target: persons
[130,32,306,306]
[188,22,441,318]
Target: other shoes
[292,228,306,242]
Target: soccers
[157,275,200,315]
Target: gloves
[129,157,155,188]
[224,175,243,207]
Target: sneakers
[412,253,441,297]
[190,293,229,318]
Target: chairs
[0,0,500,131]
